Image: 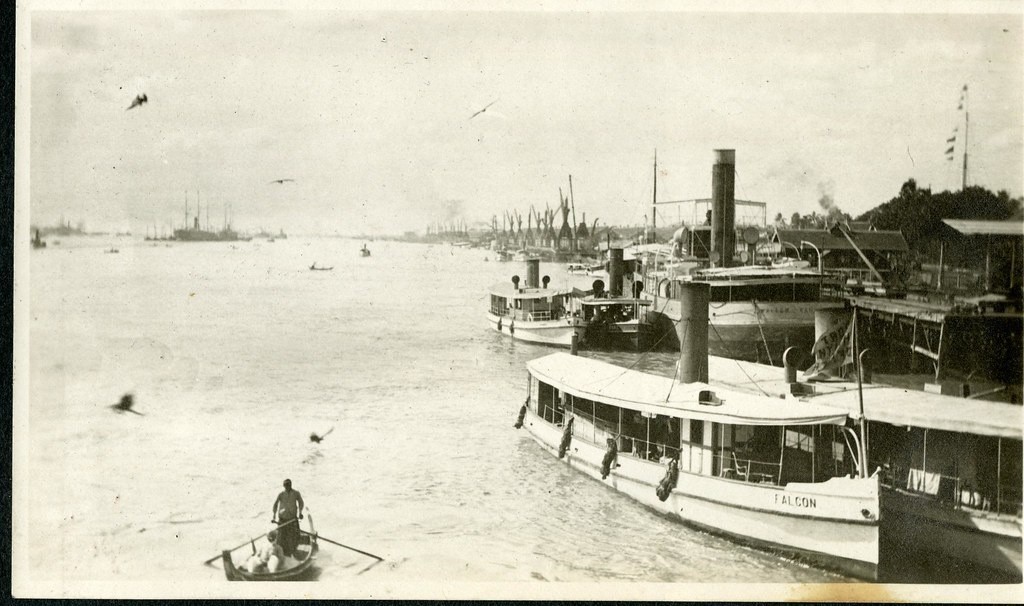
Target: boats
[496,251,513,262]
[521,352,880,583]
[485,287,589,349]
[221,508,317,581]
[634,225,845,371]
[359,249,370,257]
[568,263,605,278]
[579,298,655,352]
[675,355,1024,577]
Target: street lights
[800,240,820,271]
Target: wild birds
[268,178,293,184]
[470,99,495,119]
[127,93,149,110]
[109,393,145,416]
[310,426,334,443]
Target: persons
[273,479,304,556]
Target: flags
[803,312,855,376]
